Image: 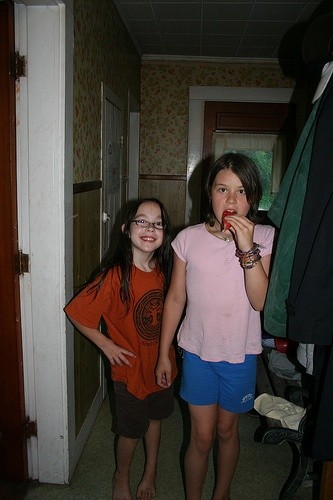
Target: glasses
[130,219,164,230]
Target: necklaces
[215,226,232,242]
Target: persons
[64,196,177,500]
[155,153,278,500]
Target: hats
[311,61,333,104]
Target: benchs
[257,347,309,500]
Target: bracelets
[235,242,261,269]
